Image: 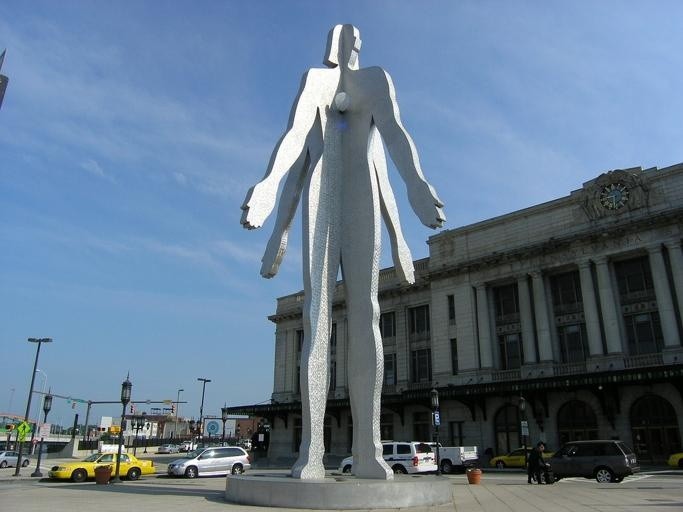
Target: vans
[338,440,438,477]
[168,445,252,479]
[244,439,253,448]
[531,439,641,483]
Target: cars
[488,446,556,470]
[48,451,157,483]
[1,449,30,469]
[158,443,180,454]
[665,452,682,470]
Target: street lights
[12,338,53,476]
[130,411,147,456]
[31,368,49,456]
[144,422,151,453]
[175,389,184,439]
[222,403,229,447]
[430,387,442,476]
[518,393,528,469]
[110,370,134,484]
[196,377,211,448]
[30,386,54,477]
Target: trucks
[181,441,199,451]
[411,441,479,474]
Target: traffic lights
[172,405,177,414]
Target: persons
[527,442,551,485]
[656,440,667,465]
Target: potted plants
[467,462,481,484]
[94,465,113,484]
